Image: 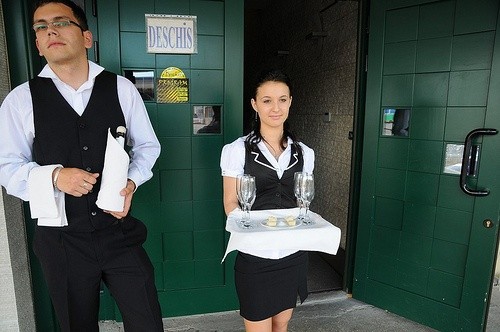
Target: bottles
[112,126,127,150]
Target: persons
[220,71,315,332]
[0,0,164,332]
[197,105,220,133]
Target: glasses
[33,19,83,33]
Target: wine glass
[236,174,256,227]
[294,171,314,225]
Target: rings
[82,181,88,189]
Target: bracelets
[53,167,63,193]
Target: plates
[261,216,302,228]
